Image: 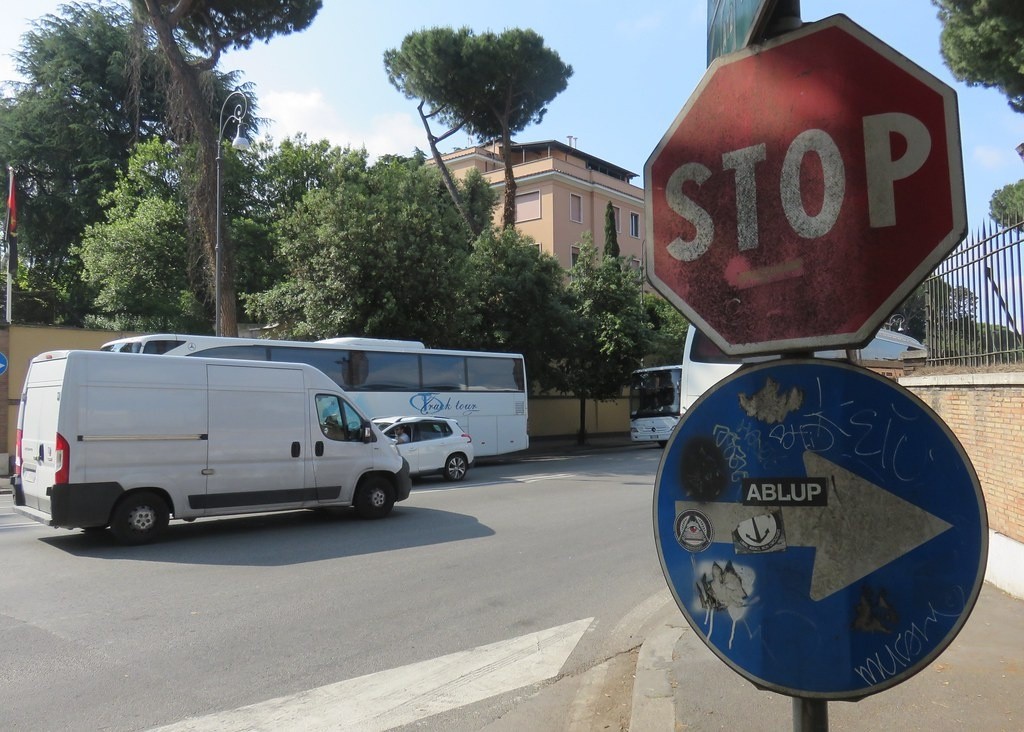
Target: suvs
[354,415,475,483]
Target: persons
[394,425,410,444]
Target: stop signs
[641,11,969,355]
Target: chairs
[402,424,421,442]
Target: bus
[99,333,530,459]
[625,365,682,449]
[677,315,927,422]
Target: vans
[8,349,413,548]
